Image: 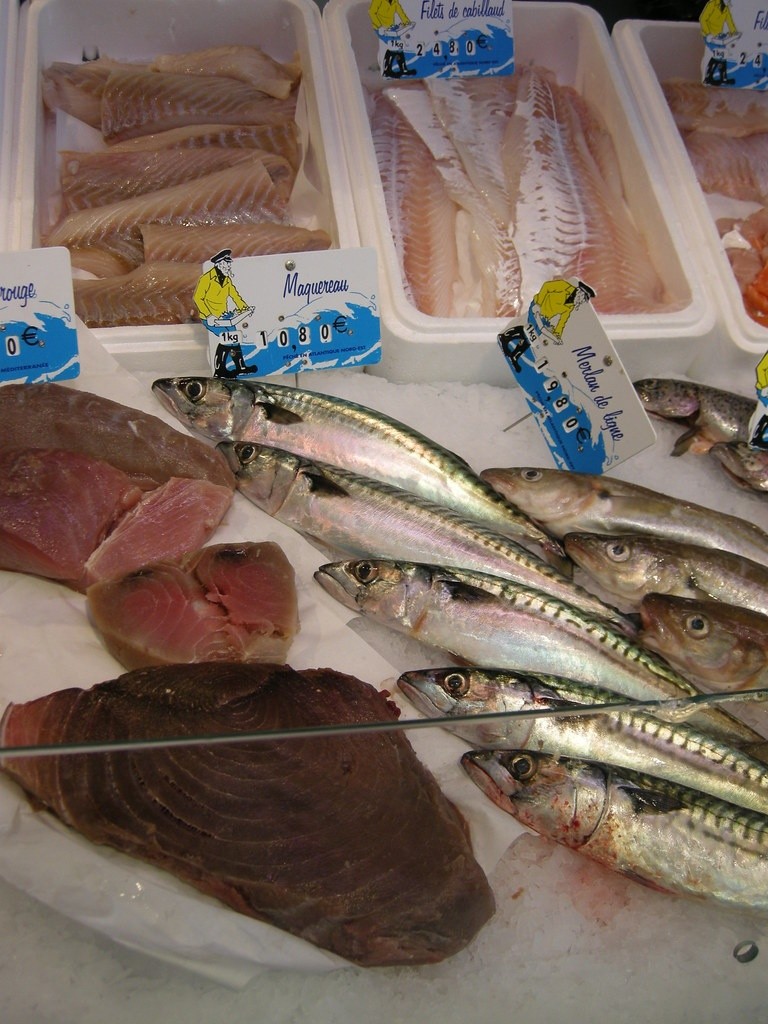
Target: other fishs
[0,44,768,968]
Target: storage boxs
[0,1,768,392]
